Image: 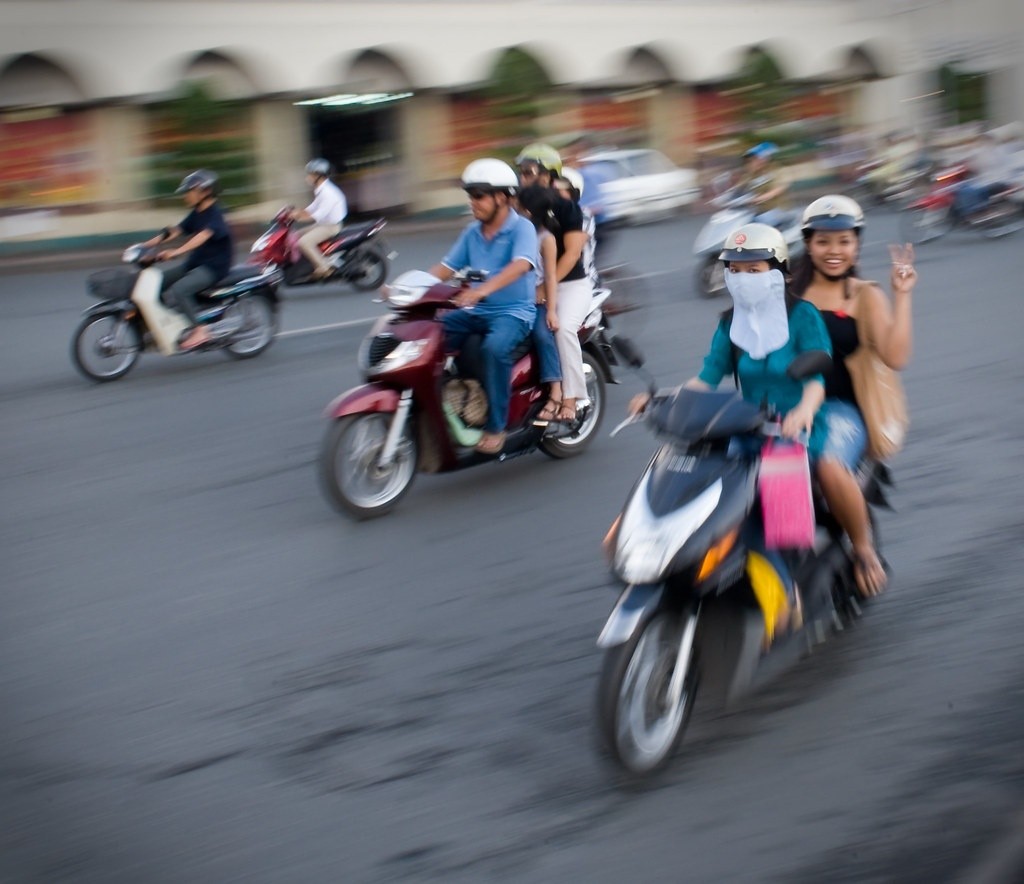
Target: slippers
[476,429,507,453]
[555,405,576,423]
[852,548,888,598]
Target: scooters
[596,336,896,782]
[692,170,808,298]
[248,203,389,293]
[316,269,649,522]
[70,226,284,384]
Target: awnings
[0,1,1024,106]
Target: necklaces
[804,285,824,311]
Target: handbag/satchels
[845,280,911,459]
[760,423,816,551]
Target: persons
[604,223,832,635]
[785,192,919,596]
[288,159,347,277]
[378,158,539,453]
[143,170,236,351]
[713,143,798,233]
[508,186,564,423]
[513,142,598,423]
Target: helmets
[801,194,865,235]
[461,158,520,196]
[718,223,790,273]
[514,142,562,179]
[740,141,779,159]
[175,171,216,194]
[305,158,331,176]
[560,166,585,200]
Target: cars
[575,149,703,227]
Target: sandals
[537,395,563,420]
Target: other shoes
[181,331,212,350]
